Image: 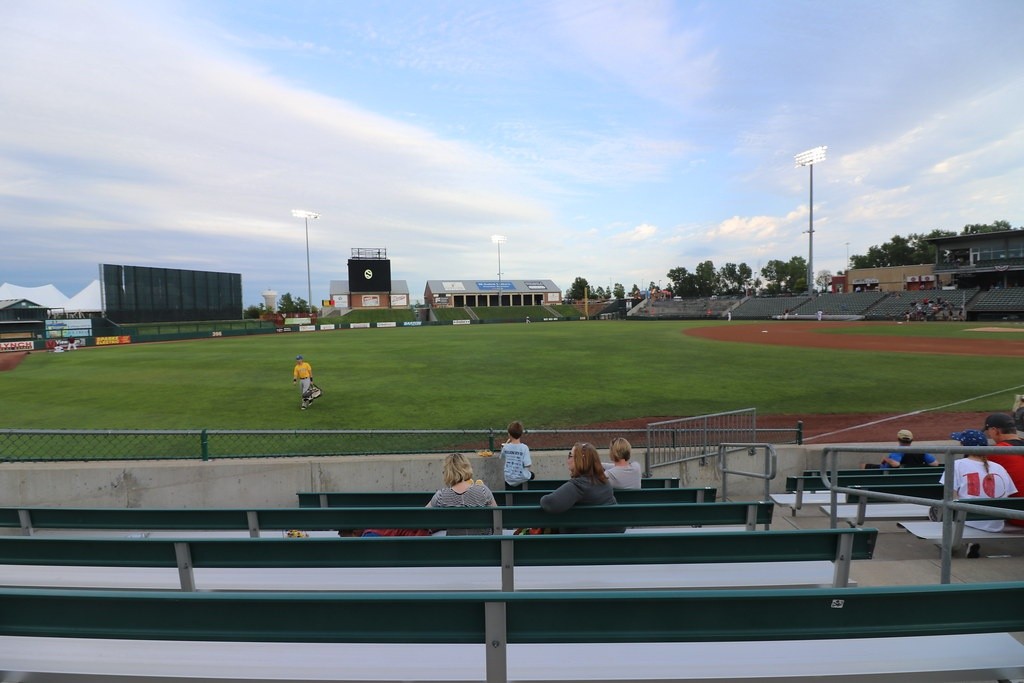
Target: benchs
[1,467,1024,683]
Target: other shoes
[301,406,306,410]
[309,401,312,405]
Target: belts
[300,377,307,379]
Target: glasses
[568,452,573,460]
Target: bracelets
[294,379,296,381]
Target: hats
[981,413,1015,432]
[296,355,302,359]
[952,429,988,446]
[897,429,913,441]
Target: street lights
[800,159,815,295]
[497,240,506,307]
[305,215,317,317]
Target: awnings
[849,282,879,284]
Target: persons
[1013,396,1024,432]
[896,284,966,323]
[855,286,861,292]
[980,413,1024,527]
[540,443,627,534]
[293,355,314,411]
[67,335,78,350]
[839,286,843,294]
[526,316,530,323]
[499,421,535,491]
[817,309,823,320]
[703,301,706,310]
[928,428,1018,559]
[601,437,642,489]
[860,430,939,475]
[727,311,731,322]
[425,454,498,536]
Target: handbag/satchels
[302,384,322,402]
